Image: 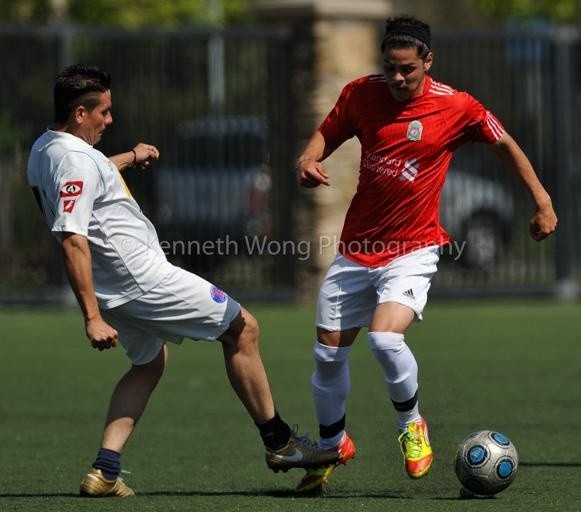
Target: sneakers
[295,431,358,495]
[265,432,339,474]
[79,466,137,498]
[398,412,435,481]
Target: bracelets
[294,158,314,170]
[130,149,139,165]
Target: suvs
[146,120,517,282]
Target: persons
[291,12,561,500]
[25,64,343,500]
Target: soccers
[454,430,518,495]
